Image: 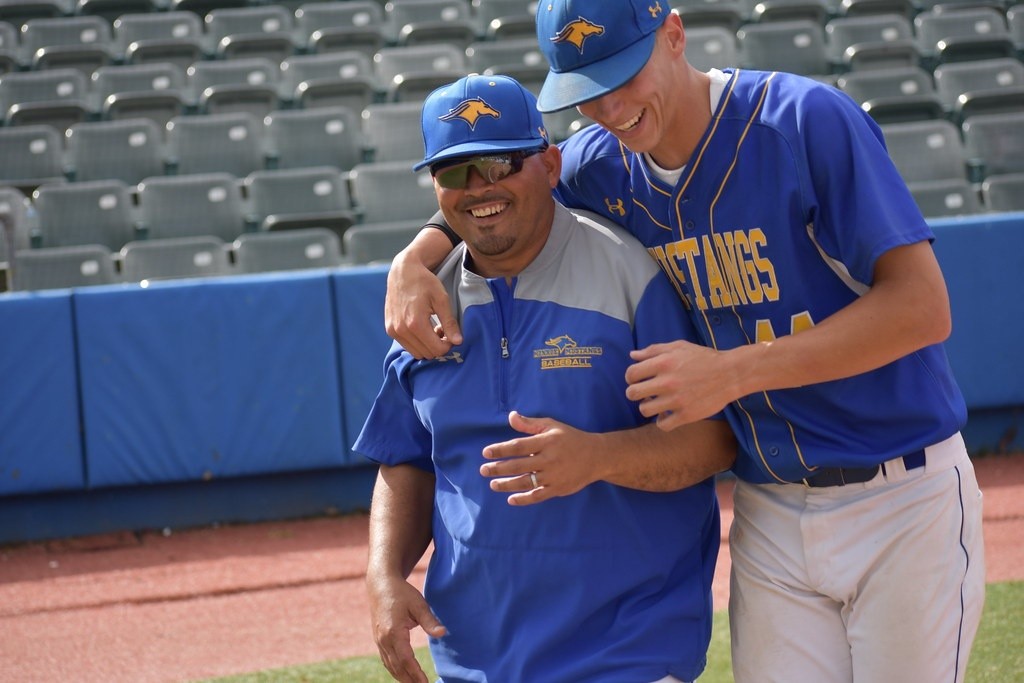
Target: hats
[412,72,548,172]
[535,1,672,113]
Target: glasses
[432,149,548,190]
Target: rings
[530,474,538,489]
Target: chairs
[0,0,1024,293]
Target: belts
[796,447,927,487]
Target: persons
[382,1,987,683]
[347,71,736,683]
